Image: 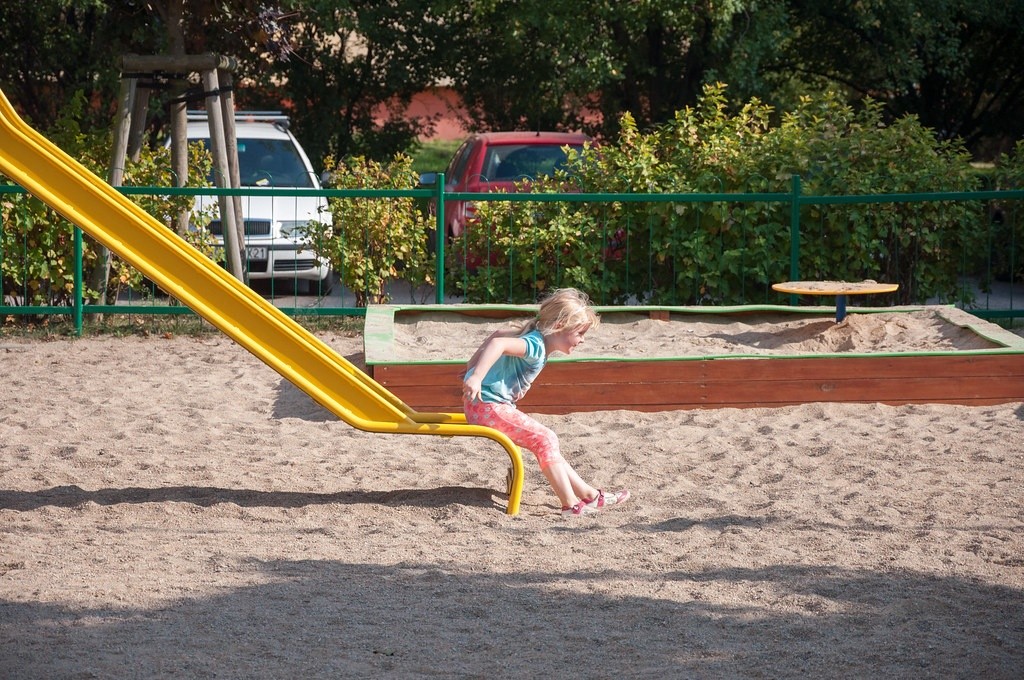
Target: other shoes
[560,501,599,517]
[581,489,632,509]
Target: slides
[0,89,527,516]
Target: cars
[418,131,629,295]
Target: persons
[456,288,630,517]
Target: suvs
[143,109,336,296]
